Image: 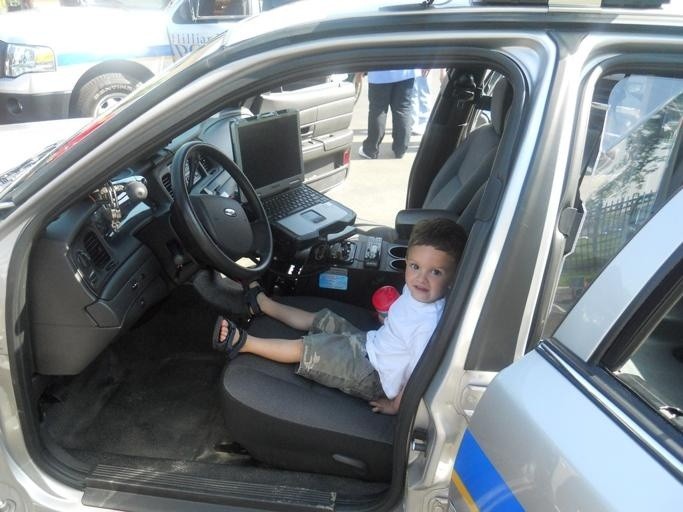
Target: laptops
[229,106,357,245]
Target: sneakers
[358,145,378,159]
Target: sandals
[241,279,267,318]
[211,314,248,359]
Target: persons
[211,215,468,418]
[420,67,448,88]
[350,65,416,161]
[411,68,431,135]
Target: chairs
[220,182,487,483]
[296,78,512,307]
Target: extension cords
[364,236,383,269]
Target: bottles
[371,285,401,323]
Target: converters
[368,245,378,261]
